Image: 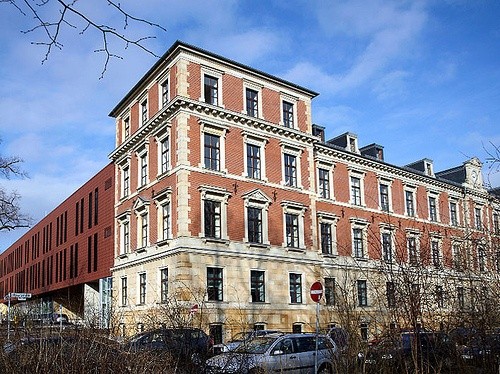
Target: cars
[0,332,119,363]
[365,327,451,360]
[50,313,68,324]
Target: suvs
[204,332,339,374]
[121,328,214,366]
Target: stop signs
[310,281,323,303]
